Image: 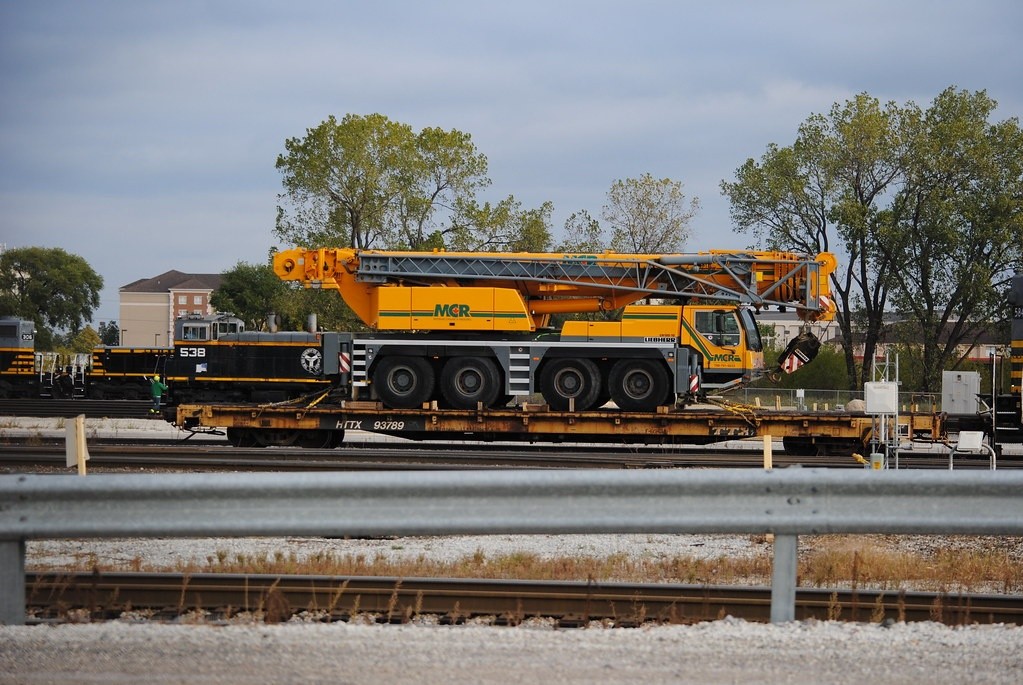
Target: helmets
[154,376,160,381]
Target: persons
[55,371,75,399]
[149,376,168,414]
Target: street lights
[155,334,160,347]
[121,329,127,346]
[167,331,170,347]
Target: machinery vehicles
[151,243,839,411]
